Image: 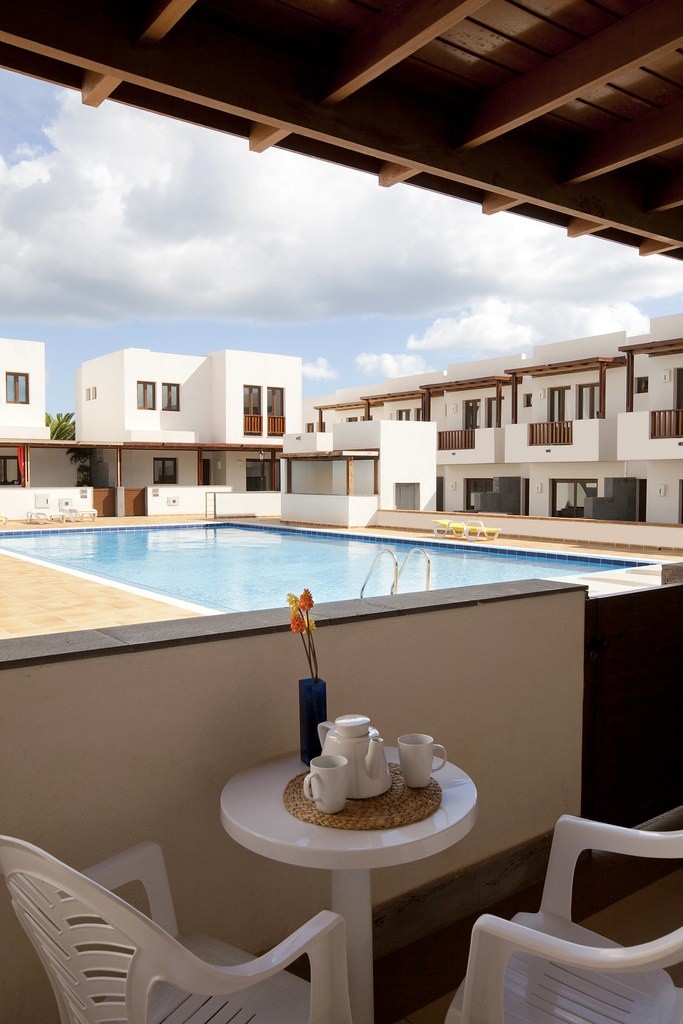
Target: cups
[302,755,350,814]
[397,734,446,788]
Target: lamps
[259,449,265,460]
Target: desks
[220,745,478,1024]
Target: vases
[299,679,326,766]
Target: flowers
[286,591,320,681]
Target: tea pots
[317,714,391,799]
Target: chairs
[61,506,97,522]
[433,518,502,541]
[445,816,683,1024]
[0,841,352,1024]
[0,511,8,525]
[26,511,67,525]
